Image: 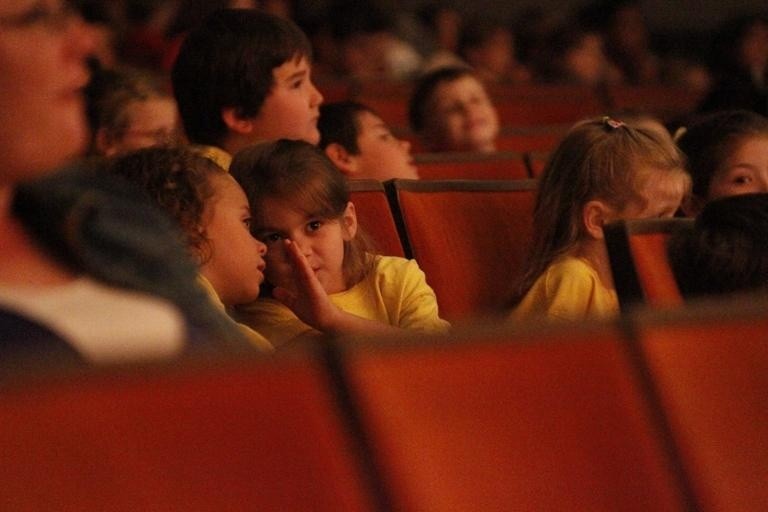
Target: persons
[6,143,274,352]
[404,65,505,159]
[167,8,326,170]
[498,109,692,326]
[674,108,768,221]
[314,97,419,184]
[81,60,182,158]
[225,134,454,348]
[0,1,187,368]
[659,186,768,301]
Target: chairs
[383,178,538,322]
[496,127,560,154]
[623,294,768,512]
[489,82,708,120]
[359,95,410,129]
[345,177,405,257]
[411,150,531,179]
[1,333,382,512]
[526,152,549,178]
[331,323,623,510]
